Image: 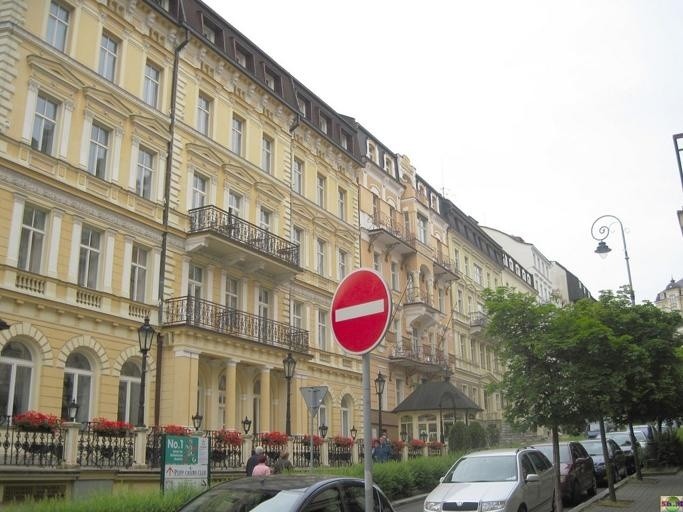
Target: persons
[250,455,270,476]
[371,436,388,462]
[245,445,267,475]
[372,439,380,454]
[272,450,292,474]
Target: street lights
[283,353,296,436]
[591,215,635,307]
[134,319,154,427]
[374,374,385,439]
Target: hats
[255,446,289,460]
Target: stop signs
[331,267,391,356]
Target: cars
[176,474,396,512]
[424,420,676,512]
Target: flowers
[214,429,244,445]
[164,424,190,435]
[304,435,323,446]
[335,437,352,447]
[14,410,60,429]
[93,418,137,434]
[261,432,286,445]
[371,439,443,450]
